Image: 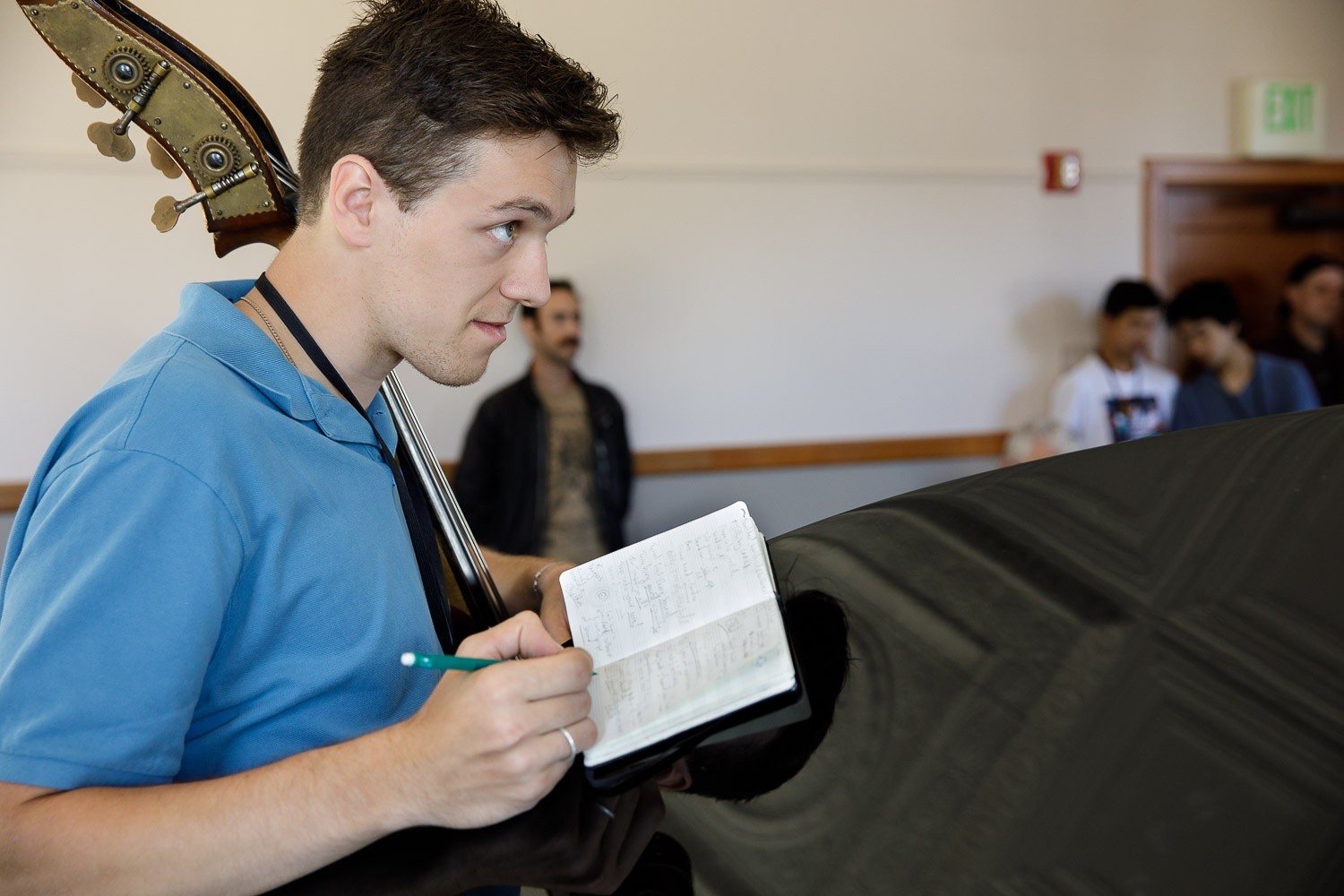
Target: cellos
[16,0,511,658]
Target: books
[559,501,796,767]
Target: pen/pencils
[400,652,598,676]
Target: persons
[1053,253,1344,455]
[1,1,619,896]
[325,590,852,896]
[453,278,633,560]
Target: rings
[595,800,615,820]
[560,728,576,759]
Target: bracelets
[532,561,563,595]
